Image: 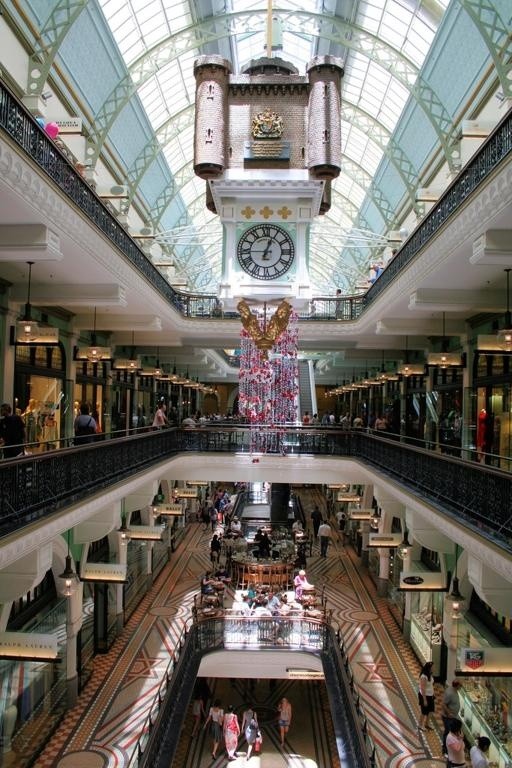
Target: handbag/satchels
[254,731,263,753]
[249,718,258,730]
[217,717,223,726]
[227,721,239,734]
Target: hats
[298,569,307,576]
[233,516,239,520]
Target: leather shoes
[212,752,217,761]
[228,752,240,761]
[418,724,435,733]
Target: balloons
[45,120,58,138]
[36,118,45,130]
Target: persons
[152,403,241,432]
[418,662,435,732]
[334,289,344,320]
[73,403,97,446]
[368,248,398,284]
[191,694,261,760]
[197,482,347,646]
[445,718,468,768]
[0,403,27,459]
[277,697,292,749]
[470,737,491,768]
[441,678,471,758]
[303,411,389,438]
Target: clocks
[237,222,295,282]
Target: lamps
[440,540,468,620]
[56,528,81,597]
[395,515,415,561]
[118,478,182,546]
[6,258,220,397]
[321,266,511,399]
[339,482,383,529]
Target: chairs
[188,519,334,637]
